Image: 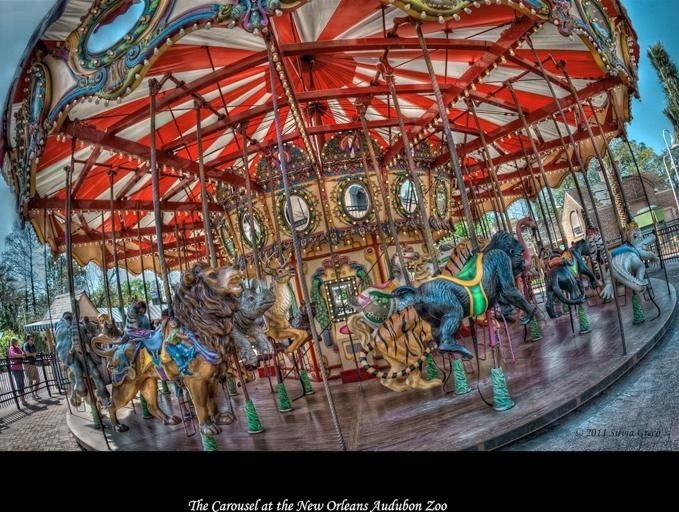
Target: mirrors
[72,0,170,77]
[390,173,428,218]
[234,206,268,251]
[435,180,449,220]
[329,175,383,227]
[324,275,360,323]
[272,187,322,238]
[219,224,237,264]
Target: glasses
[31,339,33,341]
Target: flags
[53,219,669,436]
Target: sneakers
[32,394,40,401]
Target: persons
[137,300,150,329]
[9,333,41,405]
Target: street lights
[660,129,679,216]
